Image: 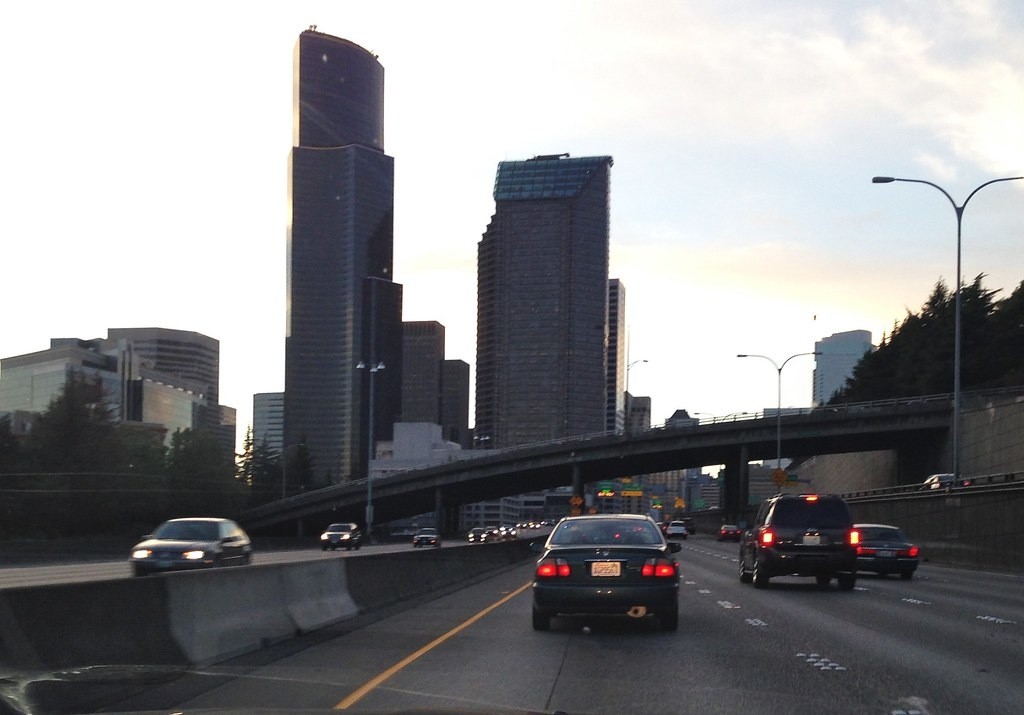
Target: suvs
[738,492,860,592]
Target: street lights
[739,353,825,491]
[473,433,490,525]
[871,177,1023,485]
[625,359,649,432]
[356,361,386,529]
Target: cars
[413,527,441,548]
[851,523,919,579]
[654,518,696,540]
[128,516,252,577]
[919,474,968,491]
[531,515,682,634]
[320,523,363,551]
[718,526,740,541]
[467,521,547,542]
